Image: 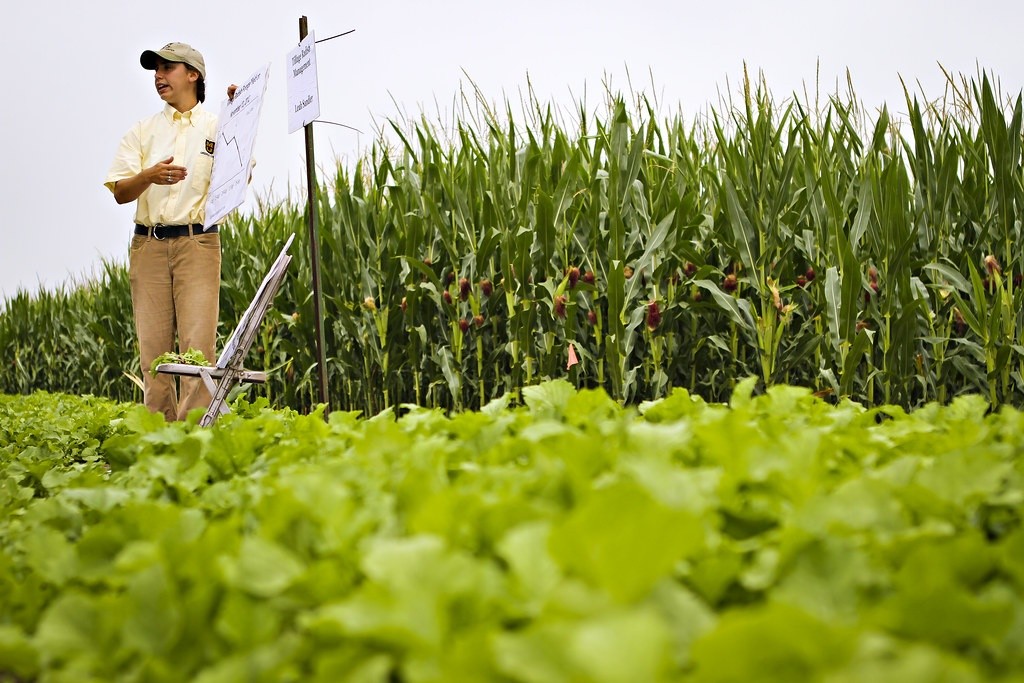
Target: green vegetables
[148,346,211,379]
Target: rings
[167,170,172,181]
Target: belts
[134,223,218,241]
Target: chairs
[155,232,296,428]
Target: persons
[104,42,257,423]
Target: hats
[140,42,206,81]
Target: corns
[363,255,1010,354]
